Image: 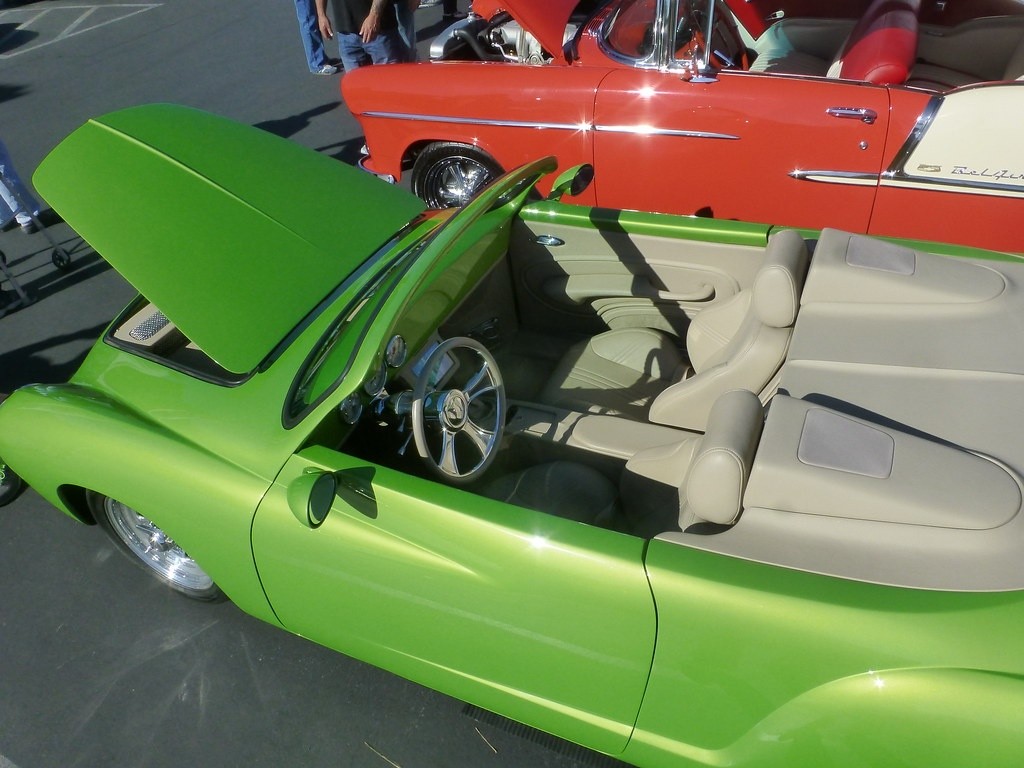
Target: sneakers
[314,64,338,76]
[325,58,343,67]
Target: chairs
[748,0,925,86]
[480,387,764,536]
[906,35,1024,91]
[528,229,809,434]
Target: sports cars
[1,104,1023,764]
[339,0,1023,262]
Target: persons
[293,0,421,76]
[0,141,40,235]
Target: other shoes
[417,0,441,8]
[442,12,468,19]
[1,217,20,232]
[20,214,54,234]
